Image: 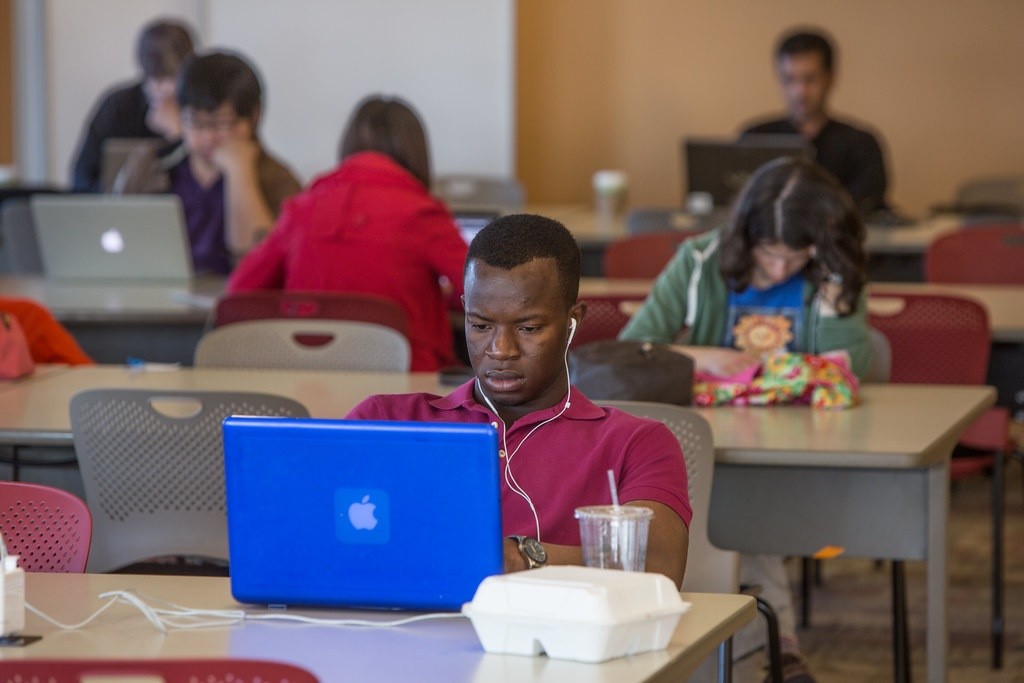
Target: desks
[0,479,94,573]
[68,386,312,575]
[508,199,965,285]
[573,274,1024,415]
[0,365,996,683]
[0,570,755,683]
[0,273,222,367]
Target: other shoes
[774,639,814,683]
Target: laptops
[27,195,195,282]
[450,209,499,248]
[221,415,504,609]
[105,139,152,189]
[683,136,816,210]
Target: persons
[109,53,303,275]
[344,214,693,591]
[72,23,194,193]
[618,155,873,683]
[225,97,468,373]
[744,32,887,210]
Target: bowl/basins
[462,565,692,664]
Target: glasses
[180,106,247,131]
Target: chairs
[429,167,526,216]
[569,211,1024,683]
[194,288,413,372]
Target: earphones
[567,318,576,344]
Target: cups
[594,170,626,222]
[575,505,653,572]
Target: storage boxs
[459,566,691,663]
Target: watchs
[508,535,548,569]
